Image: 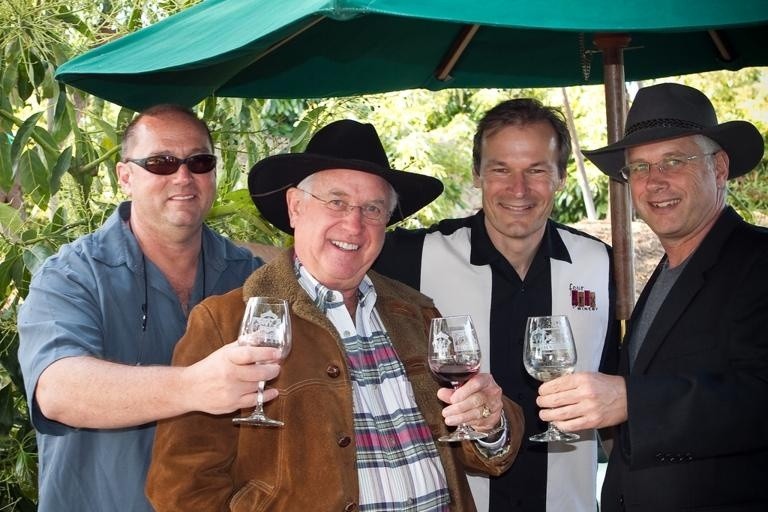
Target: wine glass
[427,314,488,442]
[522,314,581,443]
[231,296,293,429]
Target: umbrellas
[54,1,768,322]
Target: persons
[534,84,767,510]
[370,99,622,511]
[14,99,283,511]
[141,119,527,512]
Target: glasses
[296,185,393,223]
[616,152,717,183]
[121,153,219,175]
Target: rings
[482,404,491,419]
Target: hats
[579,80,767,183]
[247,117,446,238]
